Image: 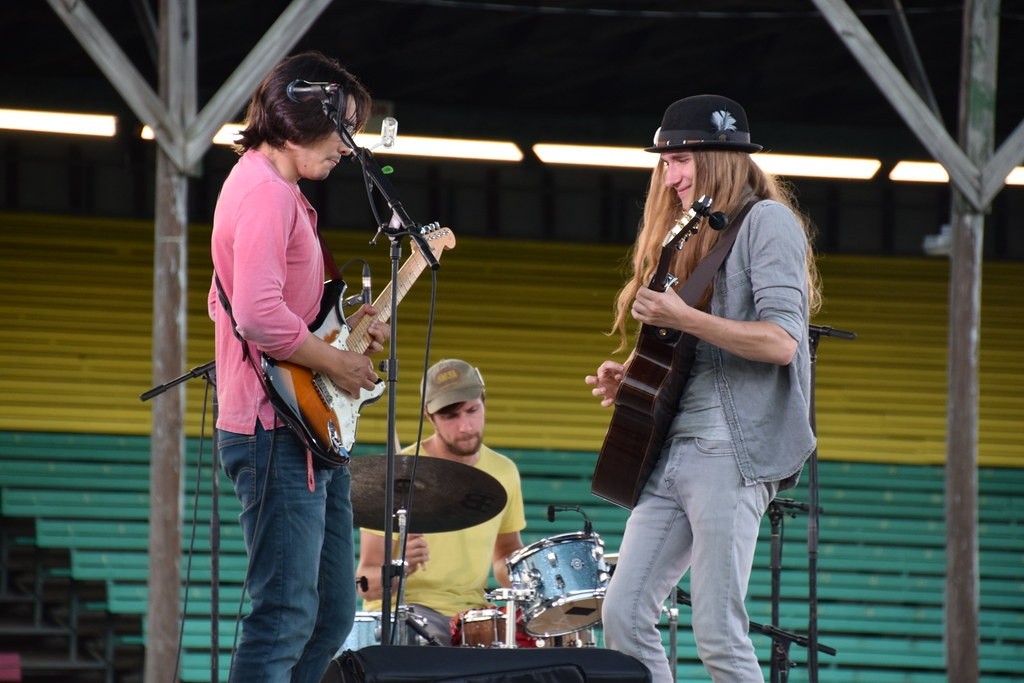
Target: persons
[207,54,388,683]
[355,360,530,645]
[584,94,817,683]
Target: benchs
[1,213,1024,683]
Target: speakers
[319,645,655,683]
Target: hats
[644,93,764,154]
[420,359,485,415]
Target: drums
[461,609,509,648]
[504,530,611,637]
[331,611,416,658]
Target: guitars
[591,195,716,513]
[260,221,455,465]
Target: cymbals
[348,456,507,533]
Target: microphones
[286,78,344,105]
[361,263,373,308]
[547,505,592,533]
